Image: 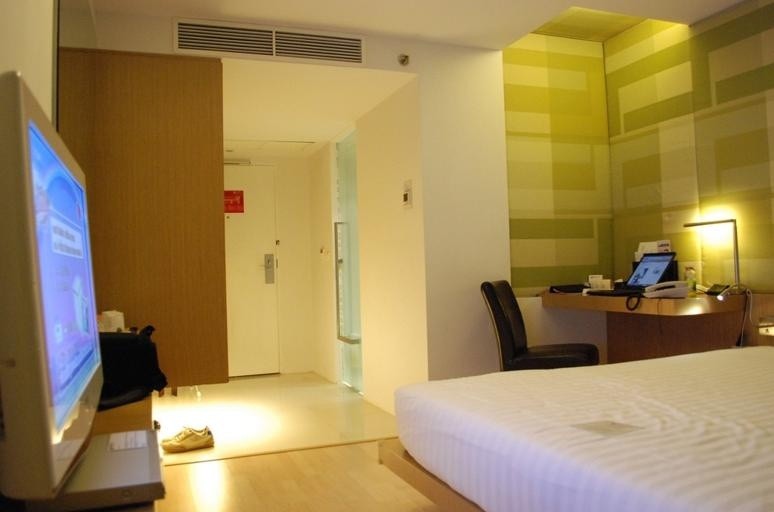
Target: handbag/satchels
[99,325,166,411]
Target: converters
[707,284,729,294]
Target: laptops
[586,252,677,294]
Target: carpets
[156,369,399,466]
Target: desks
[0,393,166,512]
[541,290,750,316]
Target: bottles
[684,266,697,297]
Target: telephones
[643,281,689,299]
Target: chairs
[480,279,599,371]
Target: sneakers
[161,426,214,453]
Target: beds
[378,345,774,512]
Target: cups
[588,275,611,290]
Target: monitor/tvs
[0,69,103,501]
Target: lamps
[717,283,754,347]
[683,218,747,295]
